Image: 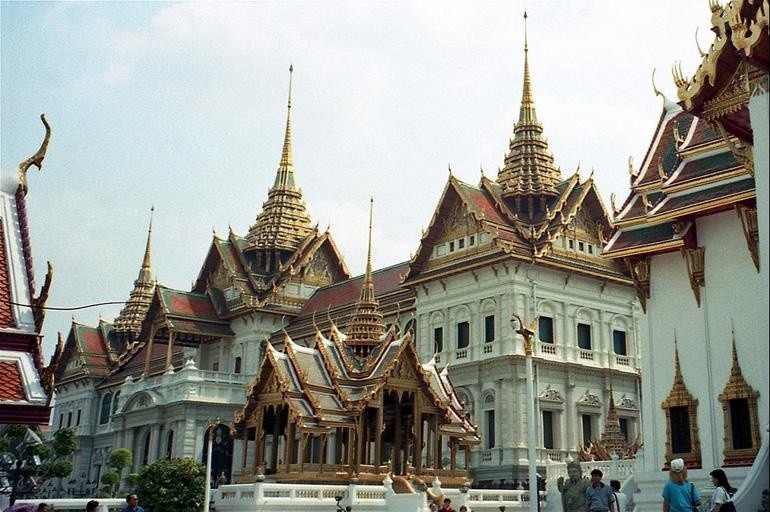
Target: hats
[671,458,684,473]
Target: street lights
[510,313,538,512]
[204,416,222,512]
[0,455,42,506]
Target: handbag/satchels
[719,487,737,512]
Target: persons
[557,459,586,511]
[36,503,49,512]
[709,469,738,512]
[585,468,627,512]
[662,458,702,512]
[121,493,145,512]
[758,489,769,511]
[428,498,469,512]
[87,500,101,512]
